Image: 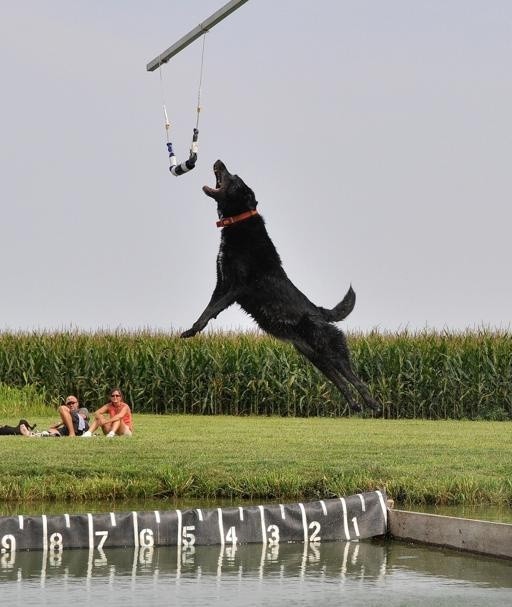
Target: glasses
[67,401,77,405]
[111,394,120,397]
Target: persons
[20,396,90,437]
[82,388,132,437]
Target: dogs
[179,158,380,412]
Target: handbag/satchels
[0,420,29,435]
